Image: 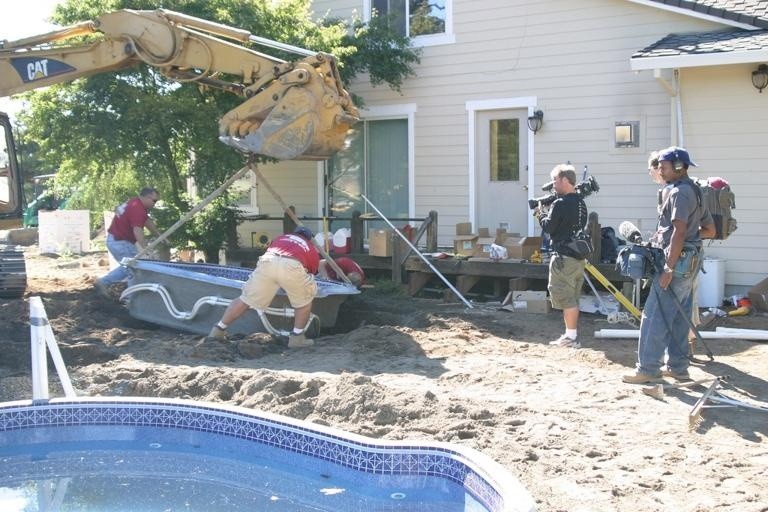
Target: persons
[252,231,271,250]
[622,148,718,382]
[533,164,587,347]
[95,188,171,299]
[646,150,699,357]
[312,257,365,288]
[206,227,319,347]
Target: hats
[294,227,312,240]
[659,147,698,167]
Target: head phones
[671,146,684,172]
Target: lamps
[750,63,767,93]
[526,110,543,135]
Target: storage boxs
[511,290,552,315]
[451,221,543,261]
[367,226,417,258]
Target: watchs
[663,263,673,273]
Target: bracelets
[535,212,541,218]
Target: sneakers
[94,278,112,300]
[288,331,314,349]
[662,366,690,380]
[210,325,226,341]
[623,371,664,384]
[549,336,581,349]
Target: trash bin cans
[693,256,726,308]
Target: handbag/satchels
[562,234,595,261]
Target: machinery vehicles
[0,2,360,302]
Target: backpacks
[691,178,737,240]
